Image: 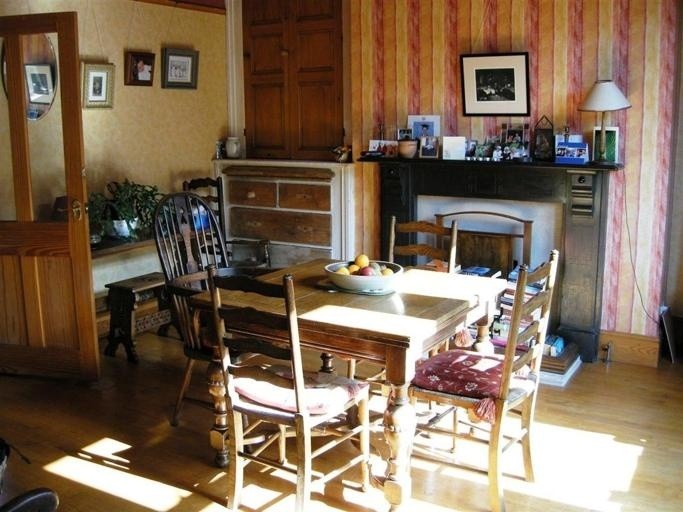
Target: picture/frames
[79,61,115,110]
[24,63,53,105]
[125,50,154,87]
[418,135,440,159]
[459,51,530,117]
[406,114,441,139]
[160,48,200,89]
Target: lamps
[576,80,633,167]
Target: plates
[359,151,384,157]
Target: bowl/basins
[396,139,417,159]
[323,259,403,292]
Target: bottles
[215,138,223,161]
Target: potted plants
[86,182,164,241]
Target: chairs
[182,176,271,270]
[204,262,373,512]
[152,192,293,427]
[345,213,458,414]
[406,248,559,512]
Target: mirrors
[0,31,59,123]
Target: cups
[224,136,241,158]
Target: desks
[186,257,509,511]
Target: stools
[102,271,184,366]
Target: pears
[336,267,349,275]
[382,269,394,275]
[349,265,359,275]
[355,255,369,268]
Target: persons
[415,124,430,149]
[508,133,521,142]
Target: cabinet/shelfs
[241,0,344,158]
[224,167,335,247]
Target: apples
[360,266,375,276]
